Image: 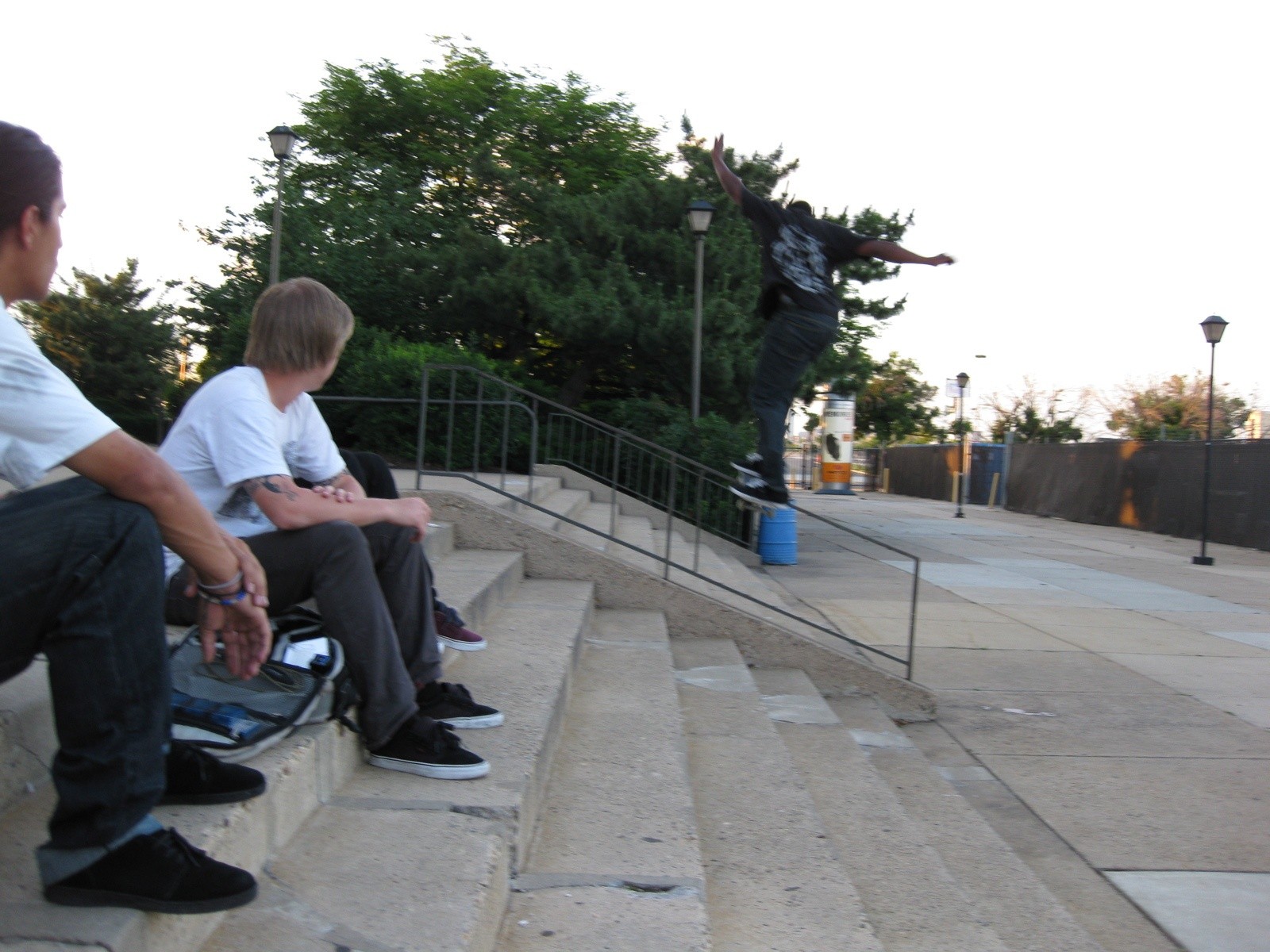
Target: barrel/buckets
[757,505,798,564]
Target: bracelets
[196,571,243,589]
[197,584,246,606]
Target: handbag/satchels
[167,603,361,763]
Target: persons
[0,119,273,915]
[711,134,953,510]
[291,448,488,655]
[155,275,506,780]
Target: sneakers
[154,739,267,805]
[433,602,487,652]
[727,479,789,510]
[729,452,764,478]
[368,720,491,780]
[415,681,504,729]
[43,826,258,915]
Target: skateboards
[727,460,776,519]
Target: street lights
[685,197,717,424]
[266,124,298,285]
[955,371,970,517]
[1192,315,1229,566]
